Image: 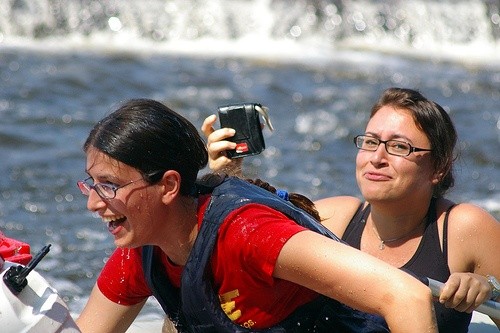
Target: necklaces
[370,215,426,250]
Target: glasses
[352,133,434,158]
[76,170,170,201]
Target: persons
[201,88,500,333]
[75,99,439,333]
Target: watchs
[487,275,500,300]
